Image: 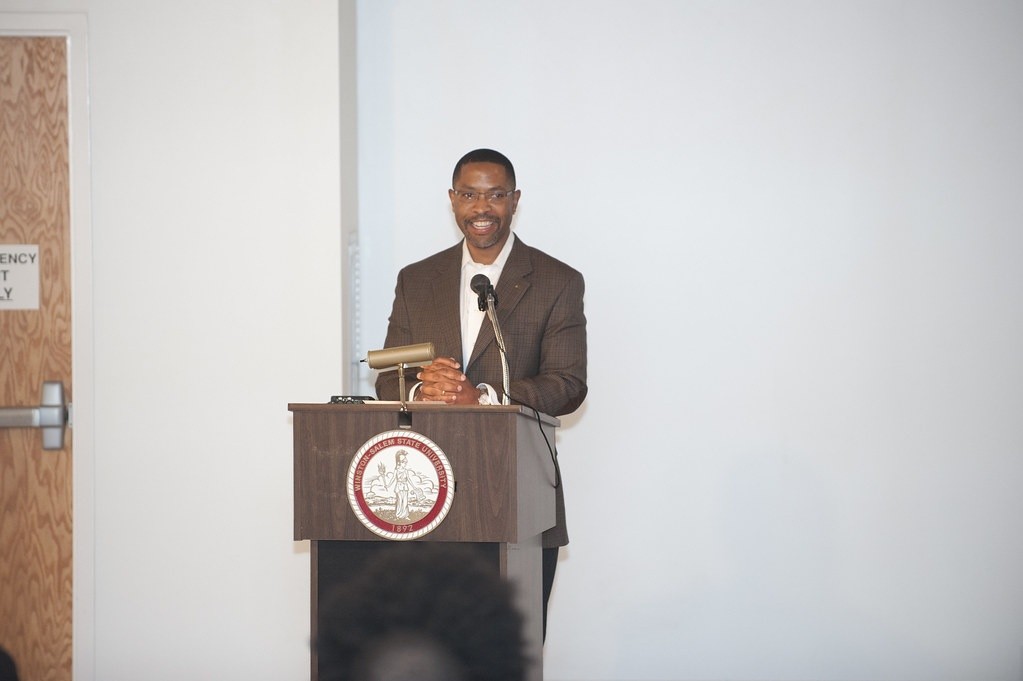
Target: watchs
[477,389,491,405]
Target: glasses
[454,189,515,202]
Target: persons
[374,148,586,649]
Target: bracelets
[413,388,422,400]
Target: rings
[441,391,445,395]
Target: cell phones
[330,395,375,404]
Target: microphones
[471,274,491,312]
